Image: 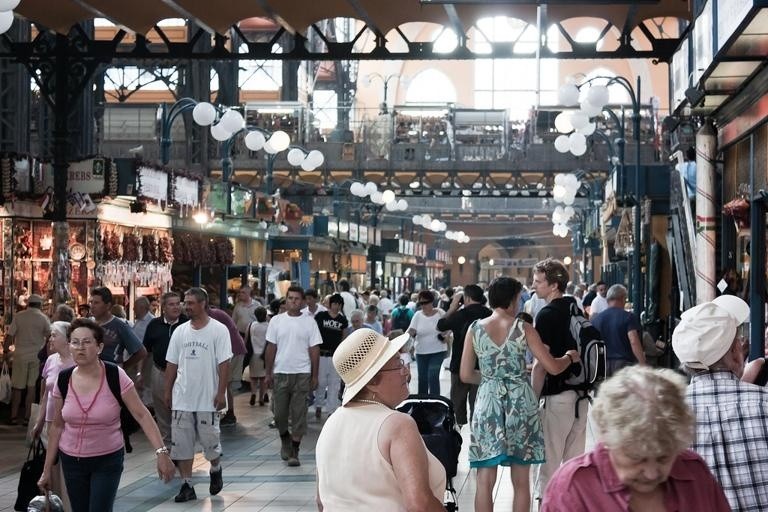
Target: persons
[460,276,581,512]
[31,321,76,512]
[163,287,232,501]
[2,283,247,451]
[530,259,608,512]
[264,287,323,467]
[539,364,734,511]
[316,329,459,512]
[670,296,767,510]
[232,280,645,427]
[38,318,175,512]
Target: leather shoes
[175,482,196,502]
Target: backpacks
[542,301,607,391]
[58,360,141,453]
[394,308,411,330]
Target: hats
[672,294,750,370]
[28,294,42,303]
[332,328,410,407]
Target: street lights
[551,69,642,328]
[457,256,464,281]
[360,71,412,108]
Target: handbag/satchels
[25,402,48,449]
[243,336,253,366]
[14,449,46,512]
[1,372,12,404]
[28,490,64,512]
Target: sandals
[4,416,29,427]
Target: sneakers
[220,415,236,426]
[278,431,300,466]
[208,466,223,495]
[250,392,269,406]
[269,418,292,427]
[315,409,321,418]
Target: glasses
[379,359,405,372]
[420,301,432,305]
[69,339,95,349]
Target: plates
[86,260,95,269]
[68,243,85,260]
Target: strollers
[393,394,462,512]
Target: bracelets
[155,446,168,454]
